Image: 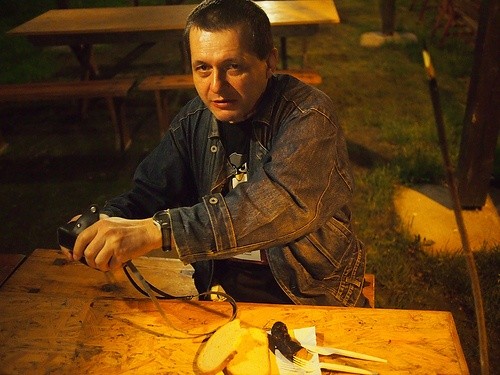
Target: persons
[60,0,368,308]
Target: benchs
[0,249,375,300]
[137,70,322,145]
[0,77,137,154]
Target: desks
[0,291,470,375]
[6,0,341,117]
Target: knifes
[301,342,388,363]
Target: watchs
[152,209,172,253]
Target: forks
[292,356,374,375]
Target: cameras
[58,221,112,272]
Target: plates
[266,327,322,375]
